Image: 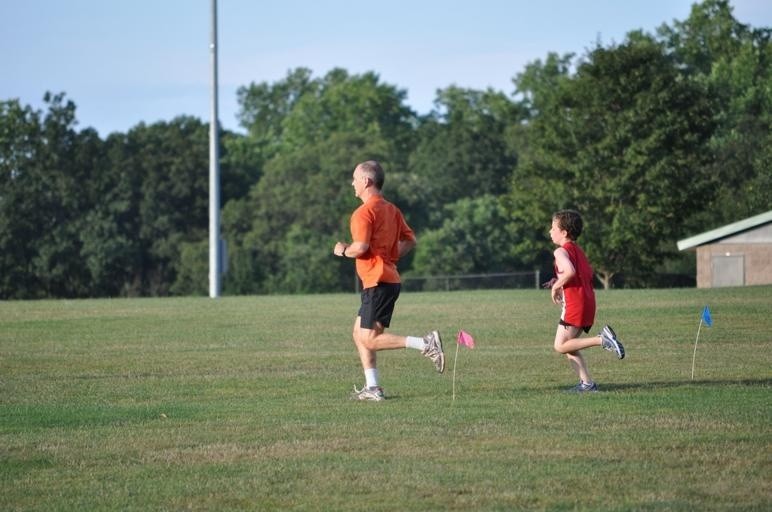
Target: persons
[335,159,446,401]
[542,209,625,388]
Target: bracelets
[341,246,346,257]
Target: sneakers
[350,385,384,401]
[598,325,624,360]
[563,380,597,392]
[421,330,444,374]
